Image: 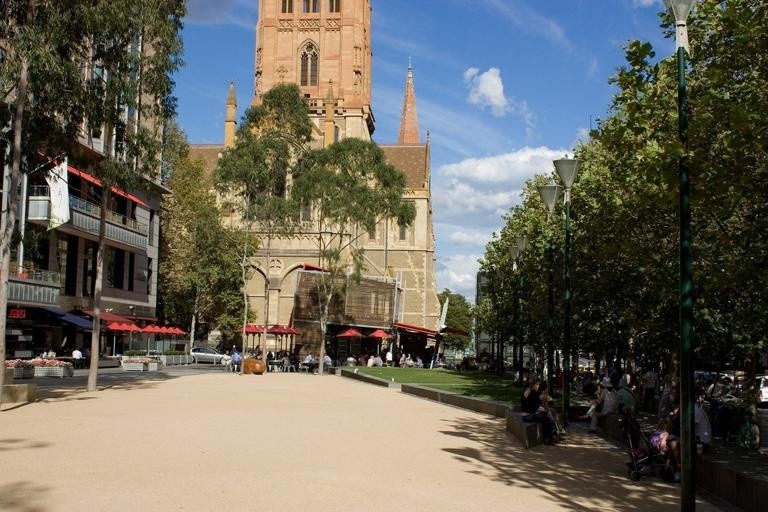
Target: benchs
[505,403,539,451]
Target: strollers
[616,402,681,484]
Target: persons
[39,346,90,369]
[458,346,712,479]
[223,344,447,374]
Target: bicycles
[725,396,761,452]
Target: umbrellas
[368,330,392,357]
[107,322,186,356]
[234,324,301,354]
[336,328,366,355]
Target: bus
[459,346,470,355]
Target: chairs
[218,356,449,375]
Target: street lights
[667,0,700,511]
[514,235,530,386]
[507,246,519,379]
[534,182,561,403]
[551,153,581,434]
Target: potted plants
[158,351,191,366]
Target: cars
[286,353,314,373]
[189,346,228,367]
[693,370,767,406]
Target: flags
[43,156,70,231]
